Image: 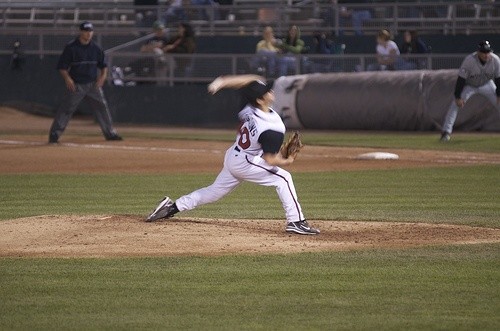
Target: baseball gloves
[281,131,303,160]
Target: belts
[235,146,240,152]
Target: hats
[478,40,492,52]
[242,78,274,100]
[79,22,94,31]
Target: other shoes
[441,132,451,141]
[48,133,60,144]
[106,135,123,141]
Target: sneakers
[286,220,321,235]
[145,195,174,222]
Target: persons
[140,20,196,86]
[48,22,122,143]
[375,28,425,71]
[257,25,331,78]
[440,40,500,141]
[144,74,320,236]
